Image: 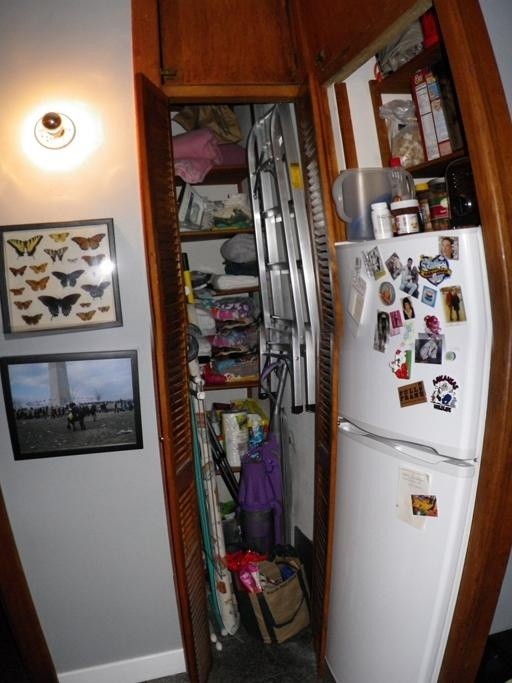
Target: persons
[367,237,461,360]
[16,398,134,434]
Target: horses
[67,406,90,432]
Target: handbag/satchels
[237,444,283,553]
[248,563,309,644]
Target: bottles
[371,177,452,240]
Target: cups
[332,168,398,241]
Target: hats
[221,234,267,275]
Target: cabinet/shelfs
[368,40,468,176]
[172,164,262,476]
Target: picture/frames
[0,349,144,461]
[0,215,125,335]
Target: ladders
[247,102,320,414]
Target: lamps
[34,108,77,150]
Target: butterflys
[7,232,111,325]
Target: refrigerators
[324,227,493,683]
